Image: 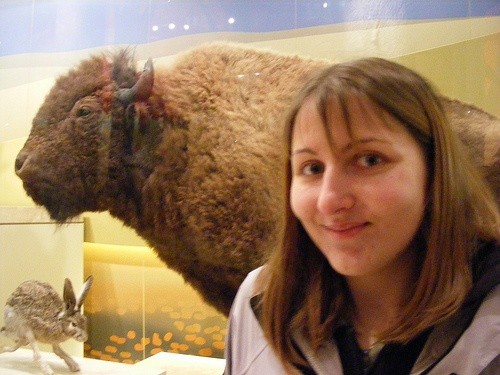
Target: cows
[14,45,500,318]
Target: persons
[221,58,500,375]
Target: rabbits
[0,275,93,375]
